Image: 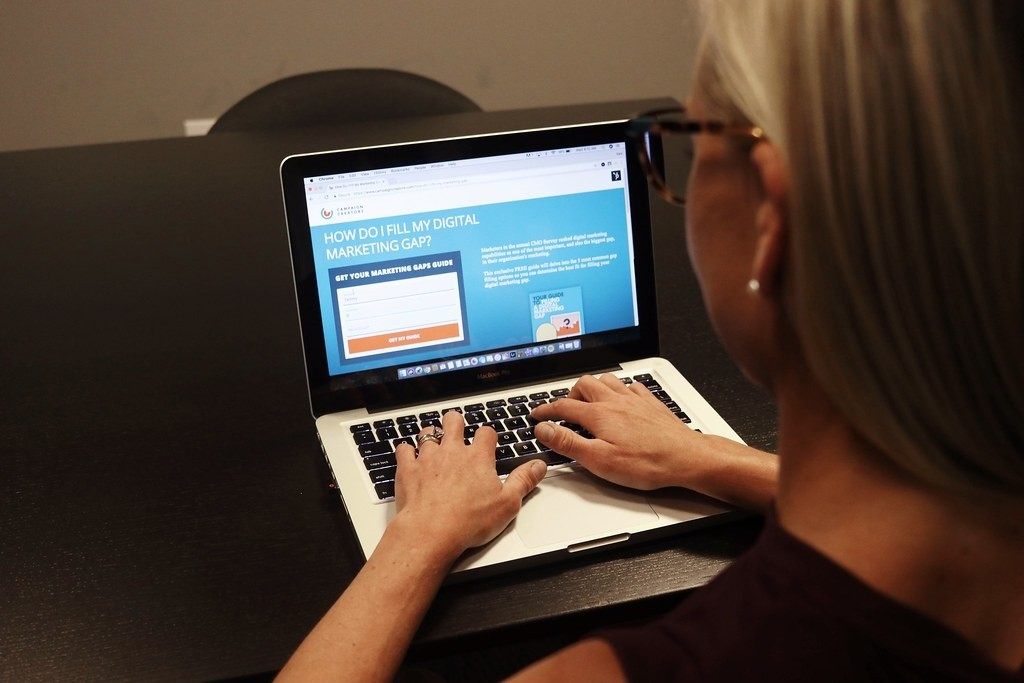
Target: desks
[0,97,778,683]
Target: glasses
[630,105,768,206]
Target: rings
[418,427,444,450]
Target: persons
[272,0,1024,683]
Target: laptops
[279,117,752,579]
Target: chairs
[207,68,480,134]
